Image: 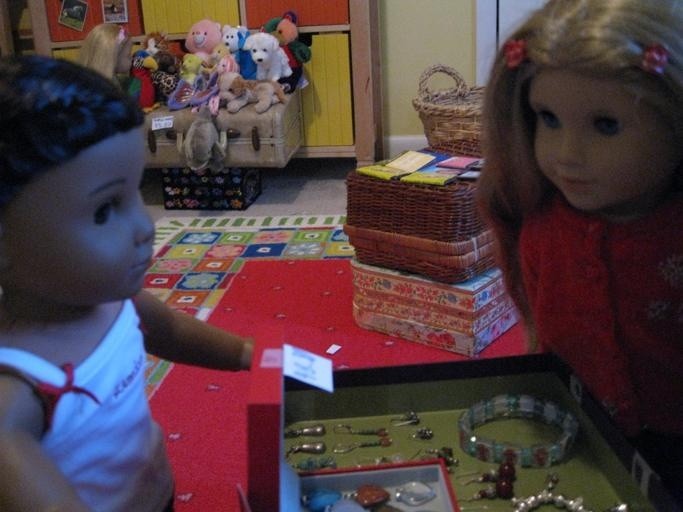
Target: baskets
[412,63,494,158]
[343,148,498,283]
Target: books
[355,150,484,187]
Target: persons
[0,53,257,512]
[78,22,133,95]
[480,0,683,450]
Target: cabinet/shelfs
[30,0,382,179]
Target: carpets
[115,210,541,510]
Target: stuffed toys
[126,12,312,177]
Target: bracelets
[510,490,586,512]
[457,392,579,467]
